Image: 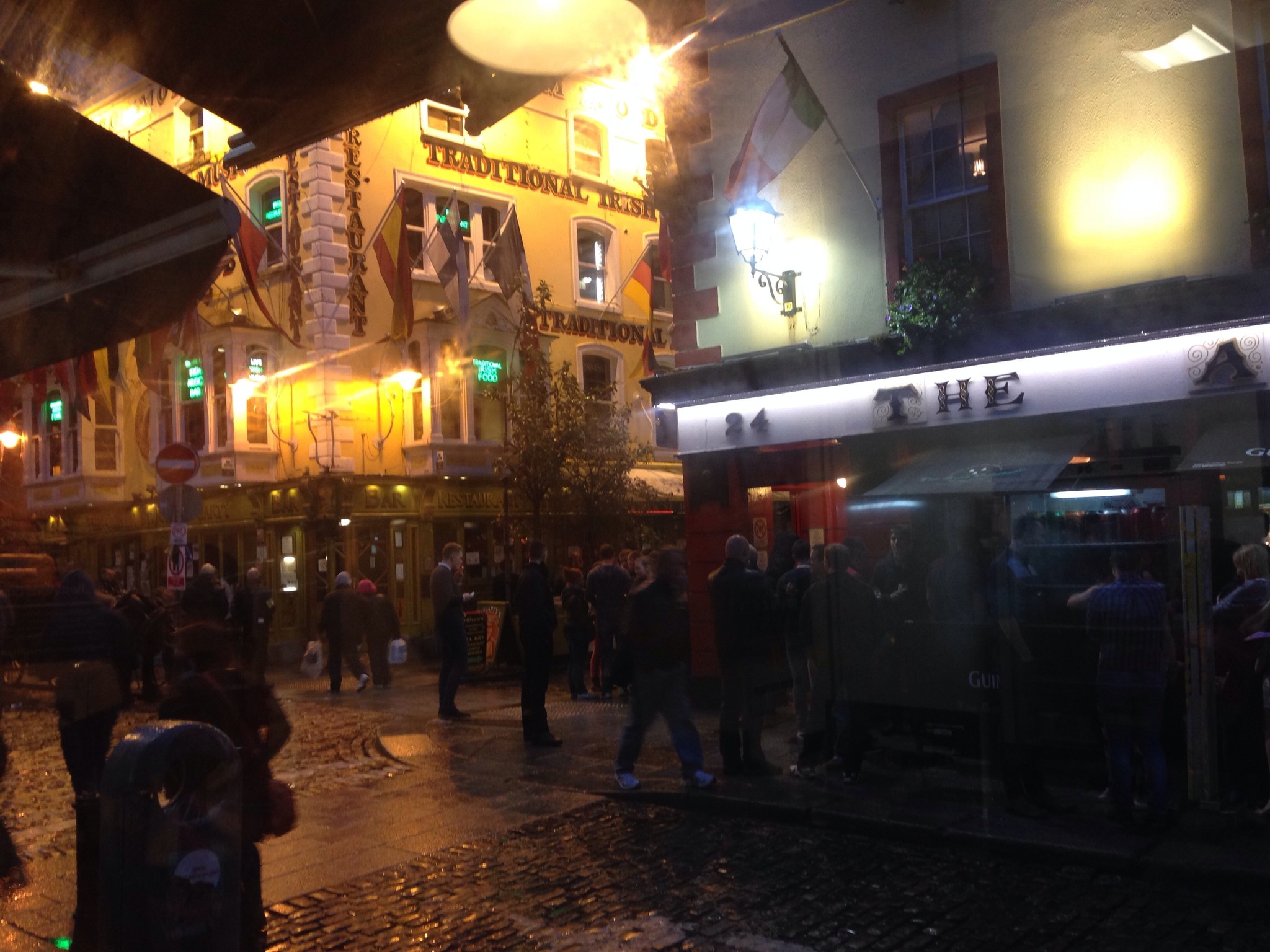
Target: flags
[218,176,294,343]
[427,194,470,337]
[619,244,659,378]
[481,208,540,355]
[724,54,824,198]
[374,187,415,343]
[0,303,201,425]
[651,209,673,283]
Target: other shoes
[796,730,806,740]
[571,693,600,701]
[724,765,744,776]
[375,680,384,689]
[790,764,823,778]
[439,707,471,720]
[602,692,613,699]
[619,690,628,699]
[0,866,26,895]
[745,761,778,776]
[841,767,860,782]
[383,675,392,685]
[525,730,563,746]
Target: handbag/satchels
[299,639,322,679]
[267,779,296,836]
[389,639,406,664]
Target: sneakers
[614,771,640,789]
[327,688,339,693]
[356,674,369,692]
[681,769,718,789]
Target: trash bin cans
[477,600,509,663]
[97,720,241,952]
[553,596,570,656]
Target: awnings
[1,0,709,380]
[861,433,1093,499]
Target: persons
[55,540,643,746]
[613,544,714,790]
[706,520,1270,774]
[66,618,292,952]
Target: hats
[173,625,227,655]
[358,578,378,593]
[334,571,352,585]
[202,563,215,574]
